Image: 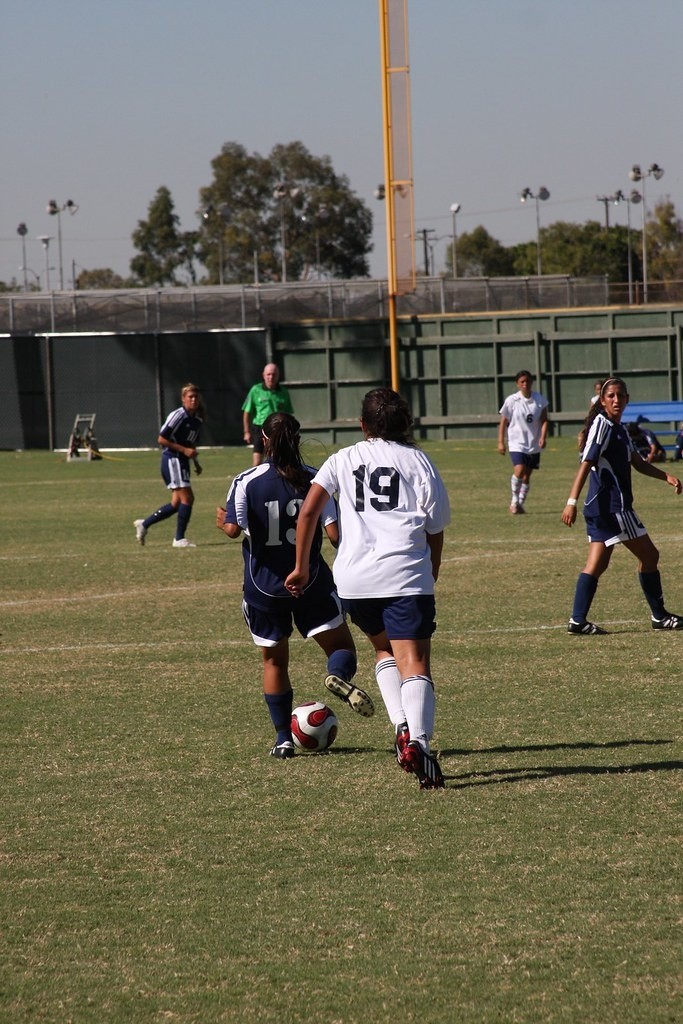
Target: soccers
[290,701,338,753]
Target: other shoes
[133,519,148,546]
[172,537,196,548]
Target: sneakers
[567,618,607,636]
[393,722,410,769]
[651,612,683,631]
[324,674,375,718]
[402,740,445,790]
[269,741,295,759]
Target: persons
[284,387,452,788]
[242,363,295,467]
[216,410,375,759]
[133,384,202,549]
[497,369,548,514]
[561,376,683,635]
[577,380,683,470]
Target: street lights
[405,234,453,277]
[520,187,551,306]
[451,204,462,276]
[628,164,664,303]
[20,266,53,287]
[614,190,642,303]
[273,187,300,282]
[47,198,79,291]
[37,234,55,291]
[17,223,29,286]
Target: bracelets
[567,498,578,506]
